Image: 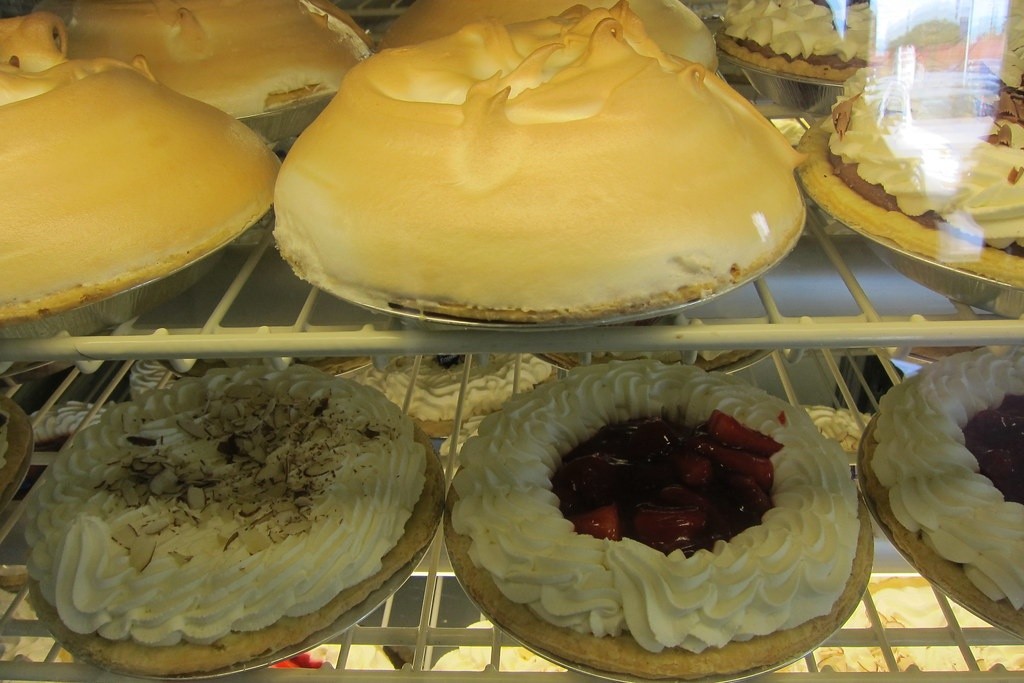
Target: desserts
[0,0,1024,331]
[0,311,1024,683]
